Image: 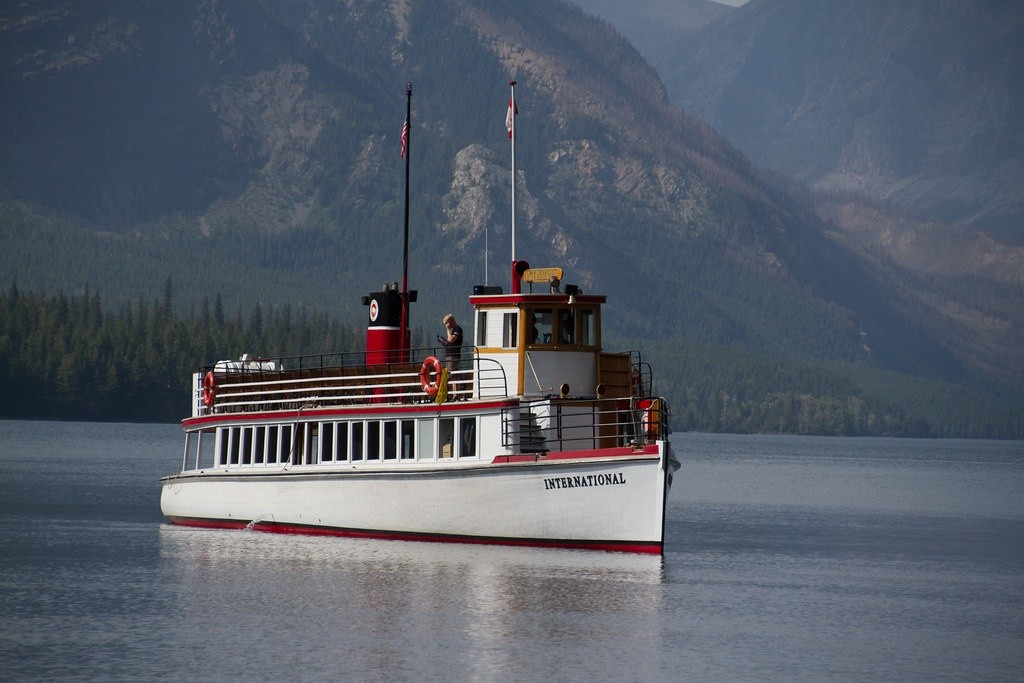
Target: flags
[505,94,518,139]
[400,112,411,160]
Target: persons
[440,314,464,401]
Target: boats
[160,77,681,553]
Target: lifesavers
[203,371,214,405]
[420,355,442,395]
